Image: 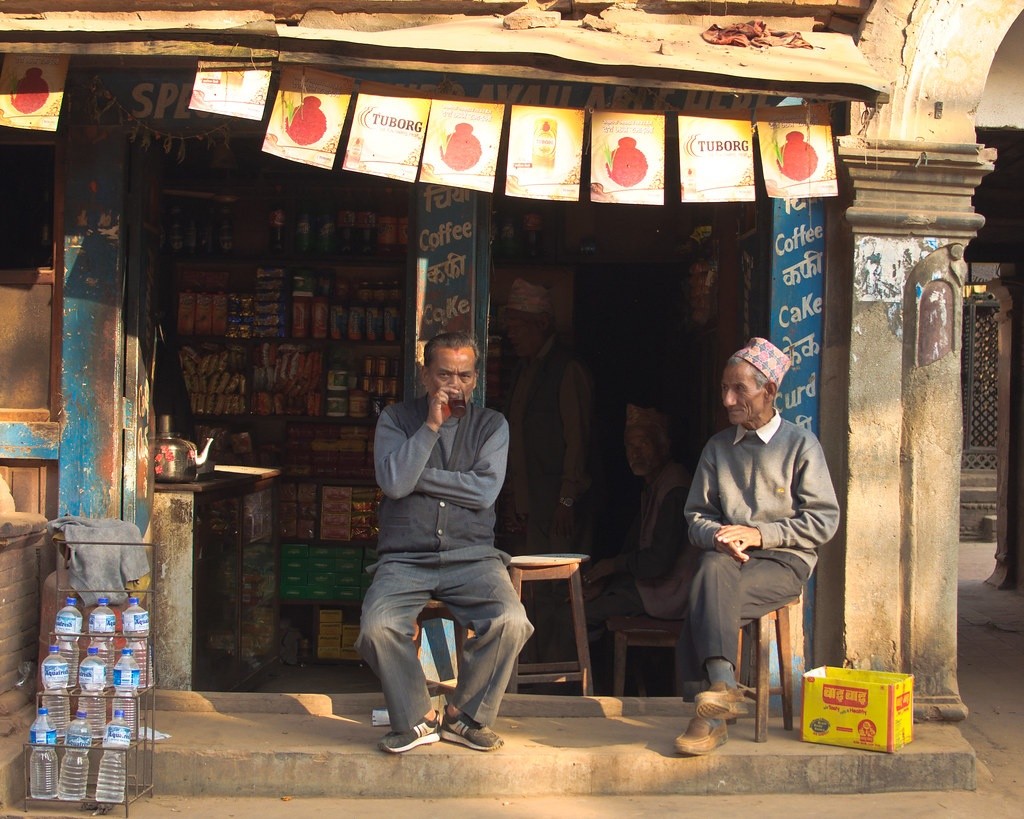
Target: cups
[448,386,466,418]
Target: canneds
[359,354,401,416]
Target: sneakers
[696,681,748,719]
[674,718,728,755]
[378,710,441,753]
[441,704,504,751]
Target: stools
[509,552,594,697]
[412,599,474,700]
[606,615,684,695]
[725,597,801,743]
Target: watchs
[559,496,577,507]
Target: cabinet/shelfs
[152,465,279,691]
[22,539,155,819]
[147,171,402,657]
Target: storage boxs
[801,665,914,754]
[283,484,364,659]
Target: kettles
[153,431,214,483]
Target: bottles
[30,597,154,804]
[159,184,544,263]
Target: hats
[736,338,792,391]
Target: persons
[496,278,602,669]
[353,333,534,755]
[529,404,700,695]
[674,337,841,756]
